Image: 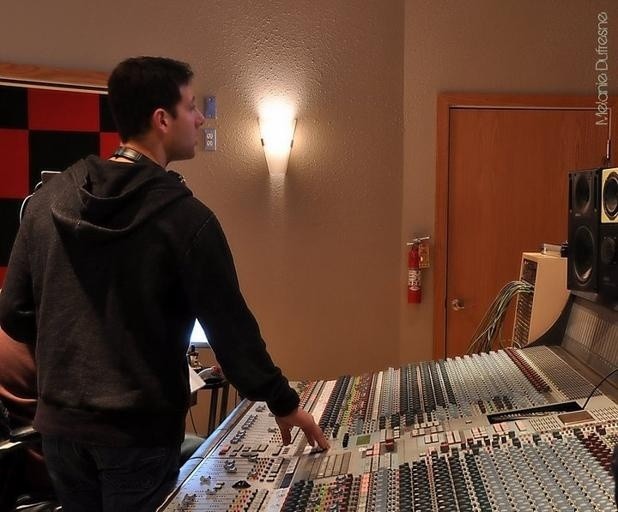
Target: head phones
[112,144,186,186]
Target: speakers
[599,224,618,288]
[566,168,600,293]
[599,167,618,224]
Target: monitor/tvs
[189,318,211,348]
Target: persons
[0,56,330,512]
[1,327,205,502]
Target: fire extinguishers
[404,236,432,305]
